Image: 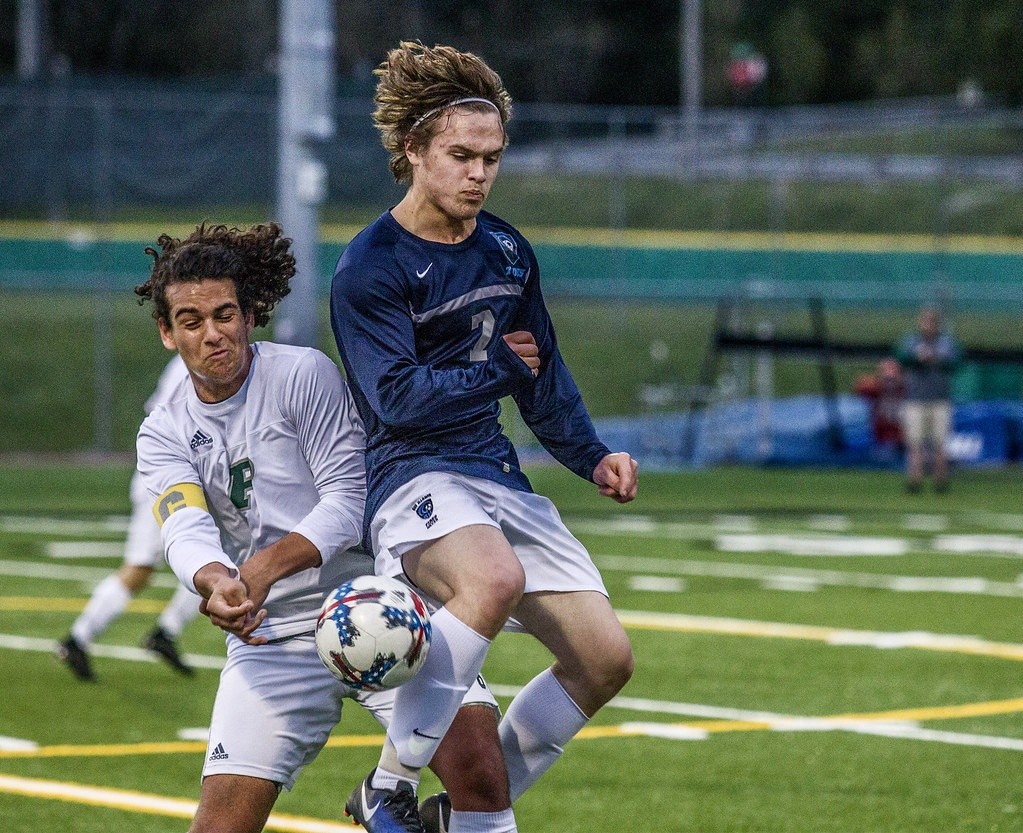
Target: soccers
[314,574,432,692]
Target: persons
[328,38,640,833]
[59,351,206,683]
[134,219,520,833]
[853,300,959,496]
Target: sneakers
[345,766,426,833]
[419,791,451,833]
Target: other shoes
[56,634,94,682]
[141,629,191,676]
